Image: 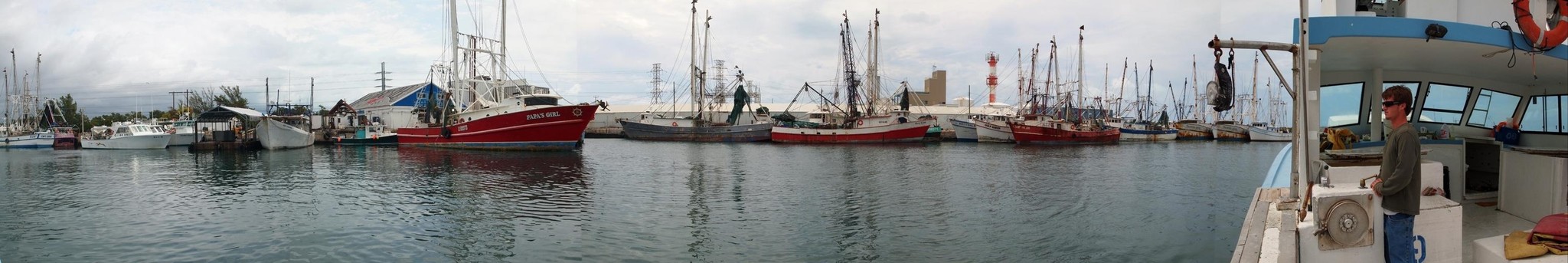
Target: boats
[974,48,1029,143]
[950,85,984,141]
[256,104,315,151]
[1103,49,1293,142]
[851,115,898,129]
[332,125,398,147]
[1229,0,1567,263]
[150,121,206,147]
[769,6,936,143]
[397,0,598,153]
[1006,26,1121,143]
[0,50,75,149]
[80,125,204,150]
[921,126,941,144]
[80,125,174,150]
[620,1,774,143]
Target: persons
[1370,85,1422,263]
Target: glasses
[1382,101,1403,107]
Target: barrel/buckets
[1382,118,1394,141]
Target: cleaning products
[1449,124,1455,140]
[1441,123,1450,140]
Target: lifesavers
[1513,0,1568,47]
